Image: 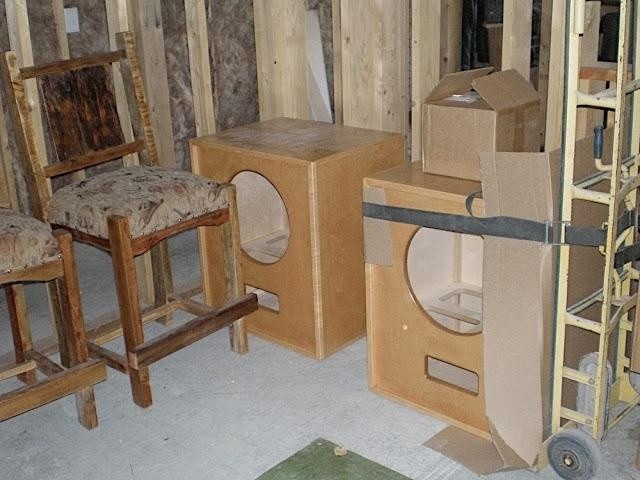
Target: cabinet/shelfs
[183,112,408,368]
[355,151,515,448]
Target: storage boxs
[412,61,548,182]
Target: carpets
[248,435,415,480]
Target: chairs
[0,103,113,433]
[0,27,264,411]
